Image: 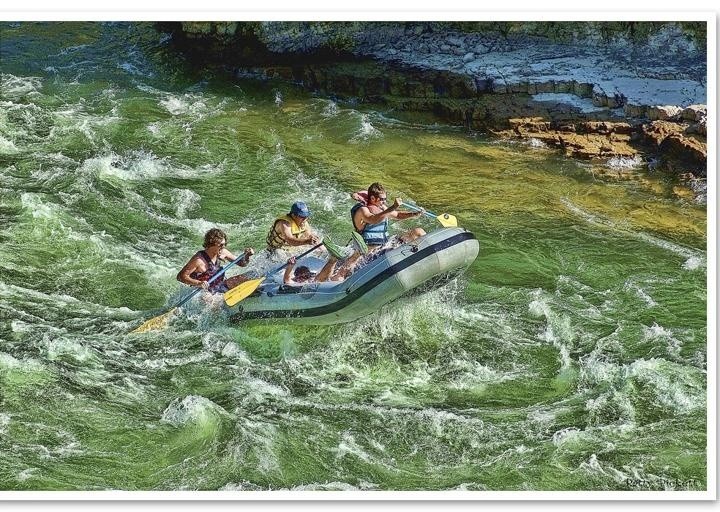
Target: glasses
[218,243,227,247]
[378,196,386,201]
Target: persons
[283,230,369,286]
[266,202,319,261]
[349,182,427,253]
[176,227,256,300]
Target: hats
[291,202,309,217]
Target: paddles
[223,242,323,306]
[131,252,247,332]
[402,203,458,227]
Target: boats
[164,224,481,329]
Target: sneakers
[351,231,368,254]
[323,238,346,260]
